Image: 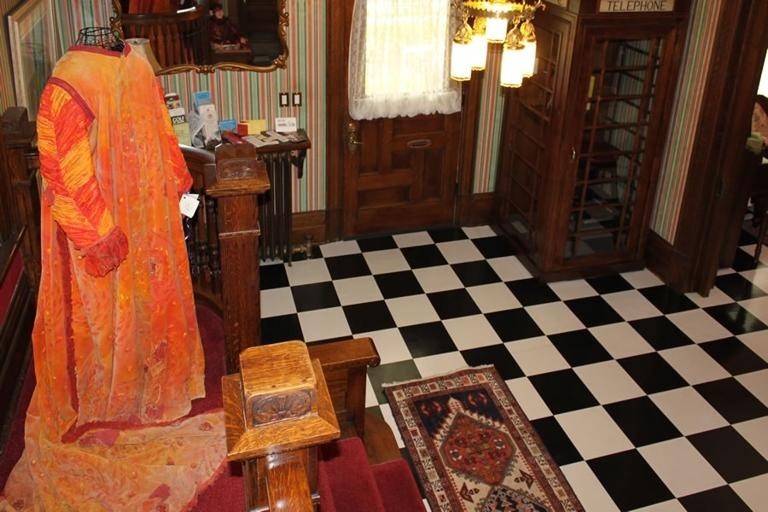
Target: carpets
[380,364,587,512]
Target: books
[221,117,309,149]
[162,90,223,147]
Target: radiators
[194,150,294,279]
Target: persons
[752,94,768,146]
[207,2,250,48]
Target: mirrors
[106,0,291,81]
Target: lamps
[446,1,540,92]
